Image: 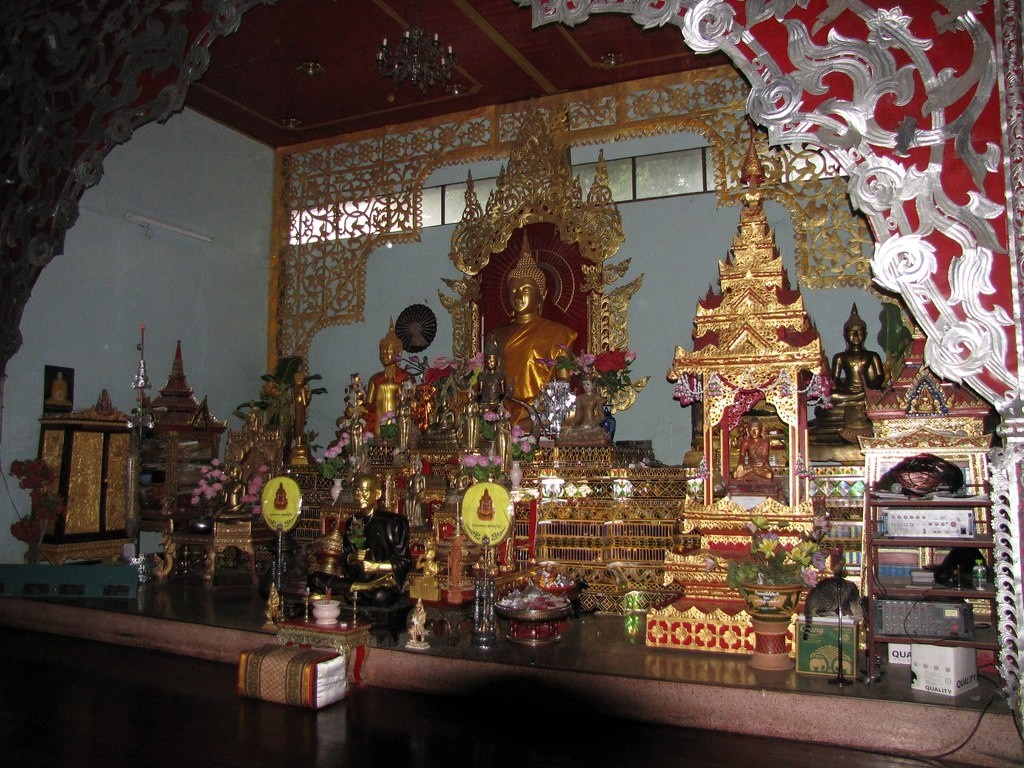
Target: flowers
[704,511,839,597]
[461,451,501,483]
[316,432,375,481]
[380,411,399,439]
[535,345,639,405]
[385,352,486,387]
[239,464,273,517]
[483,411,536,462]
[189,459,232,506]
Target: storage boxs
[794,619,860,679]
[910,644,981,696]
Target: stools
[276,616,371,689]
[153,520,259,590]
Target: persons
[366,316,416,435]
[415,551,441,581]
[375,365,405,435]
[264,586,280,619]
[493,406,513,475]
[409,600,427,647]
[96,390,116,417]
[292,372,310,448]
[220,463,246,512]
[306,474,412,610]
[561,374,606,434]
[477,354,506,411]
[45,372,73,407]
[815,302,885,421]
[732,417,774,483]
[486,229,578,431]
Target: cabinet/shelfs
[862,480,1013,689]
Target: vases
[207,501,215,518]
[599,406,616,442]
[737,581,804,672]
[509,461,523,490]
[330,478,344,505]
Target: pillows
[237,646,348,710]
